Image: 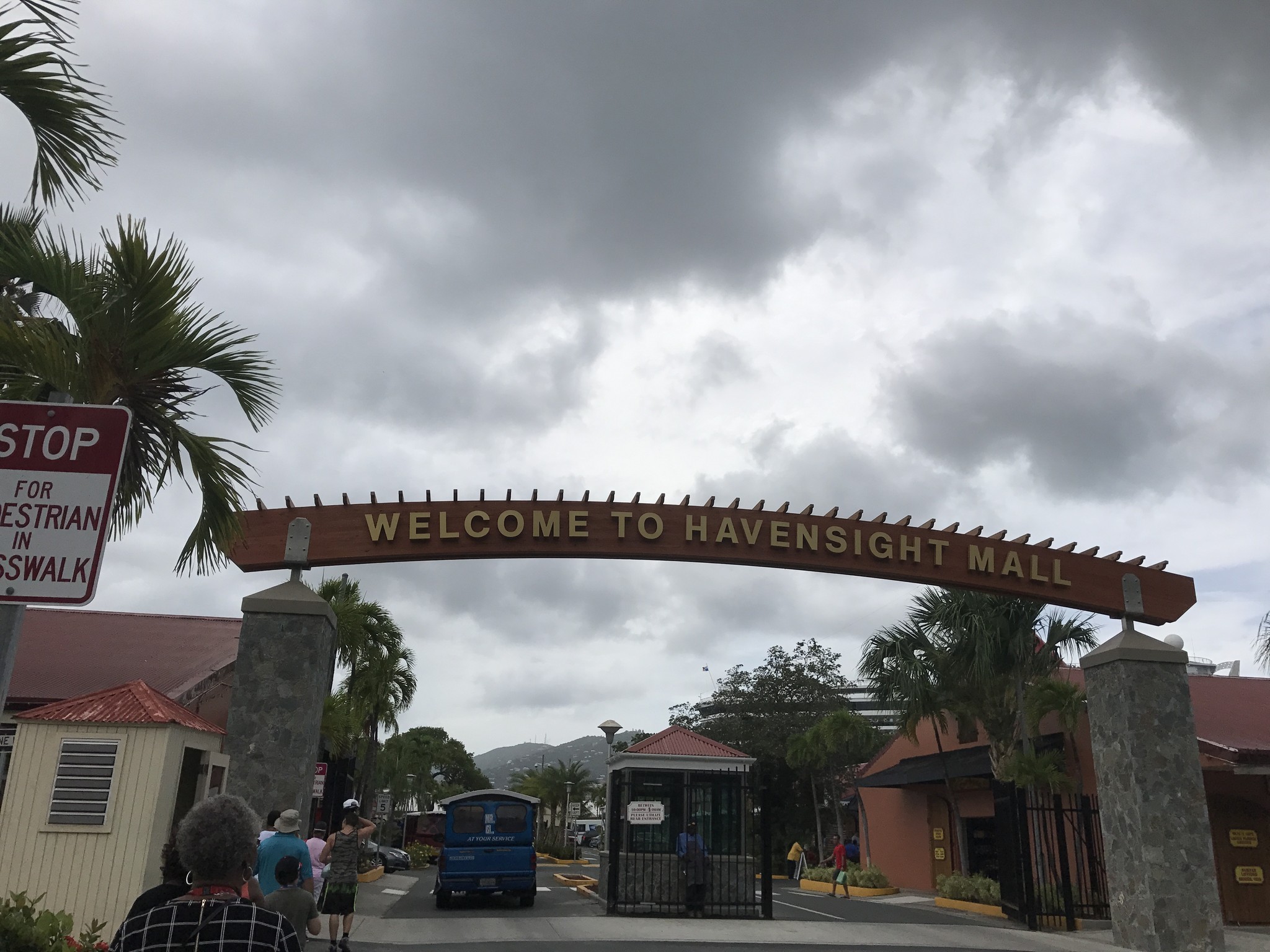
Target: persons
[108,793,377,952]
[820,834,850,899]
[788,831,860,881]
[676,819,711,918]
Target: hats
[343,799,360,809]
[274,809,300,833]
[275,854,302,879]
[314,821,326,831]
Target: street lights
[401,773,416,851]
[564,781,575,847]
[597,719,624,848]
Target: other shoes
[688,910,694,917]
[788,877,795,880]
[696,910,702,919]
[328,943,338,952]
[338,937,351,952]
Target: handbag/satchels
[836,871,847,884]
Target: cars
[361,839,411,873]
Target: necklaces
[343,828,353,832]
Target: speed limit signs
[377,794,391,813]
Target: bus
[432,790,541,909]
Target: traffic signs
[570,802,581,816]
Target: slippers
[826,893,836,897]
[840,895,850,899]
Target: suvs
[572,819,606,847]
